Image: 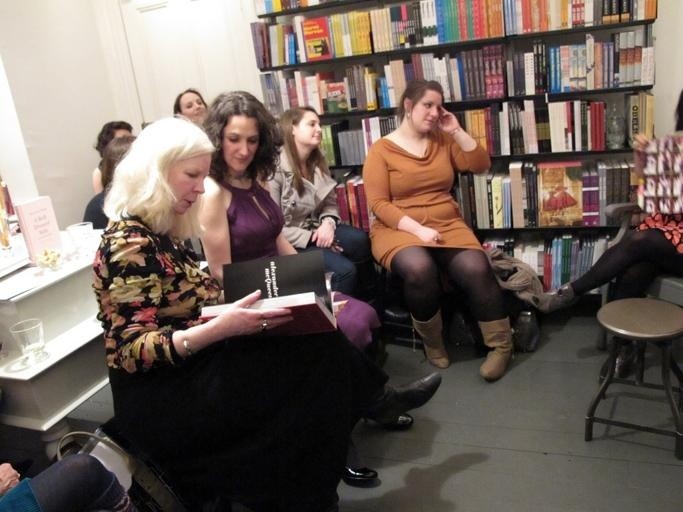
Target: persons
[264,105,388,371]
[363,79,516,381]
[91,120,134,195]
[0,454,139,511]
[92,112,442,511]
[82,135,143,230]
[185,89,414,488]
[172,89,211,125]
[540,89,683,353]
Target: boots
[599,339,636,380]
[528,281,575,315]
[477,316,514,381]
[409,312,451,369]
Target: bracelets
[180,330,194,356]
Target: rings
[260,318,271,330]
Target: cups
[66,221,93,237]
[8,318,49,364]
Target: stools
[583,298,683,459]
[646,277,682,348]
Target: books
[15,194,63,268]
[198,248,351,336]
[249,1,656,298]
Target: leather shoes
[367,371,442,429]
[395,413,413,429]
[343,462,378,480]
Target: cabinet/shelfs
[252,1,657,349]
[0,232,114,464]
